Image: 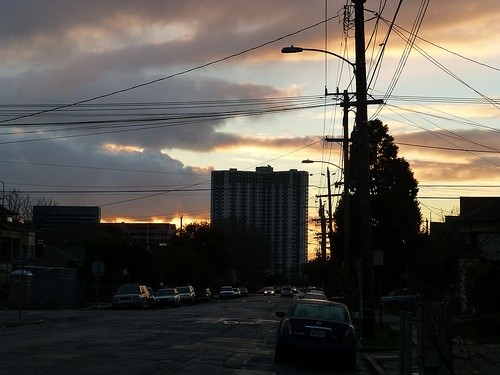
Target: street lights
[280,45,367,346]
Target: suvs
[111,284,150,309]
[175,285,196,303]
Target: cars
[233,288,241,298]
[264,287,276,296]
[281,287,293,298]
[155,288,181,308]
[381,288,421,305]
[274,299,358,368]
[256,288,264,294]
[196,288,212,302]
[146,286,155,303]
[291,287,327,302]
[219,286,235,300]
[240,288,249,297]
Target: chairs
[330,313,341,320]
[297,309,308,317]
